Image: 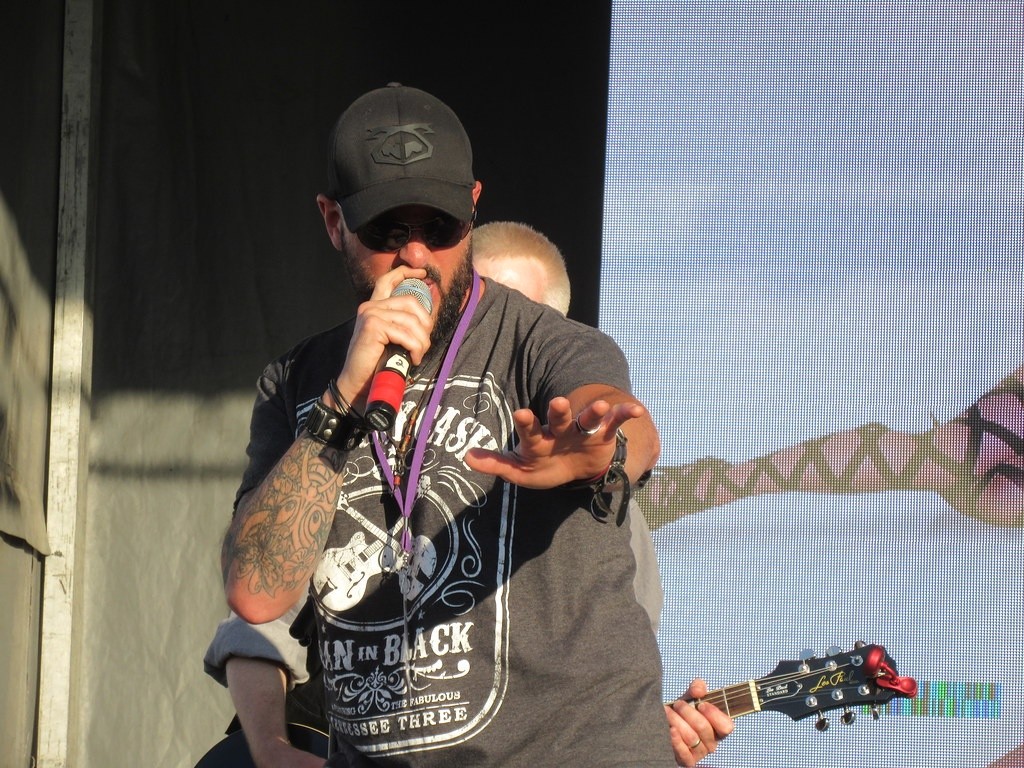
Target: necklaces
[384,321,450,497]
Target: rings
[576,413,602,435]
[690,739,701,749]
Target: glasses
[355,206,473,253]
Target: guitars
[194,640,920,768]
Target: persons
[220,79,680,768]
[634,365,1024,528]
[191,219,733,768]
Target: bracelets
[328,378,362,420]
[557,428,631,527]
[304,400,370,452]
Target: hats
[329,83,476,230]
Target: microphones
[363,278,433,433]
